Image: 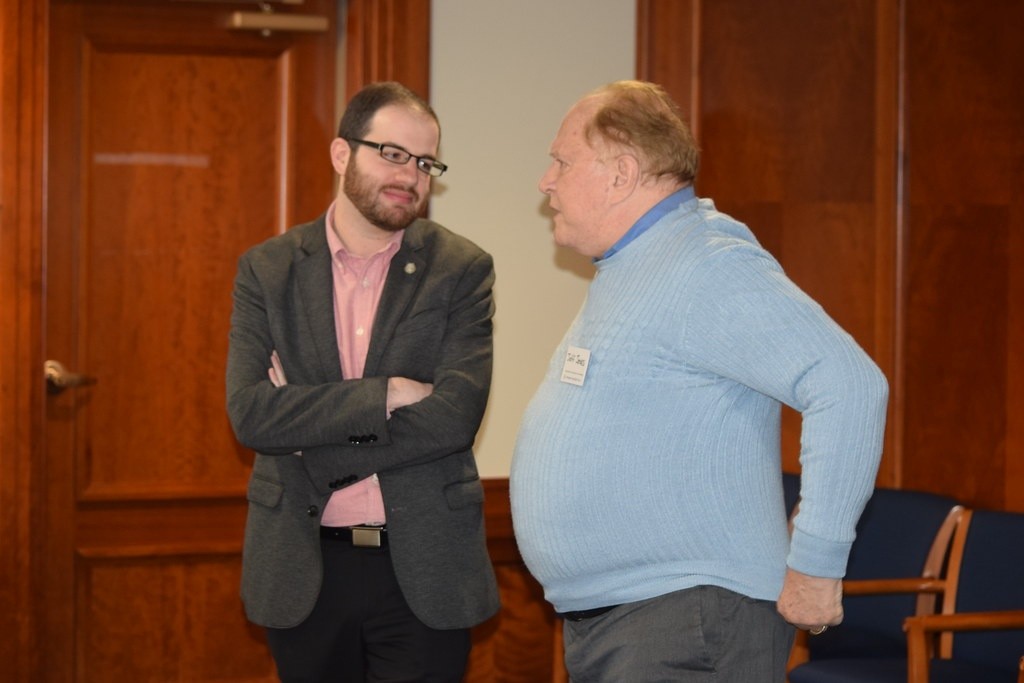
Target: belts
[319,524,388,550]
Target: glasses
[345,137,448,178]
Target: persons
[509,78,890,683]
[224,81,501,683]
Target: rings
[810,625,828,635]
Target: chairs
[784,487,965,682]
[782,507,1023,683]
